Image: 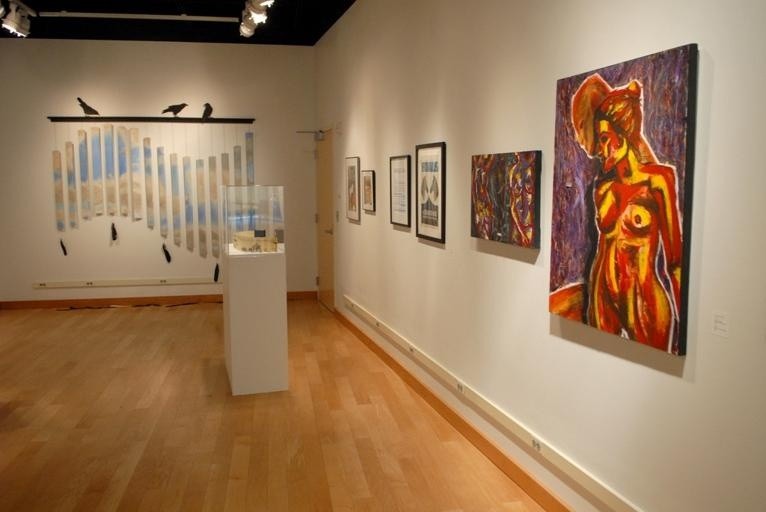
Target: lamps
[1,0,35,41]
[237,0,276,38]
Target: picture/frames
[344,141,446,244]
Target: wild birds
[76,97,100,117]
[202,102,213,121]
[161,103,189,117]
